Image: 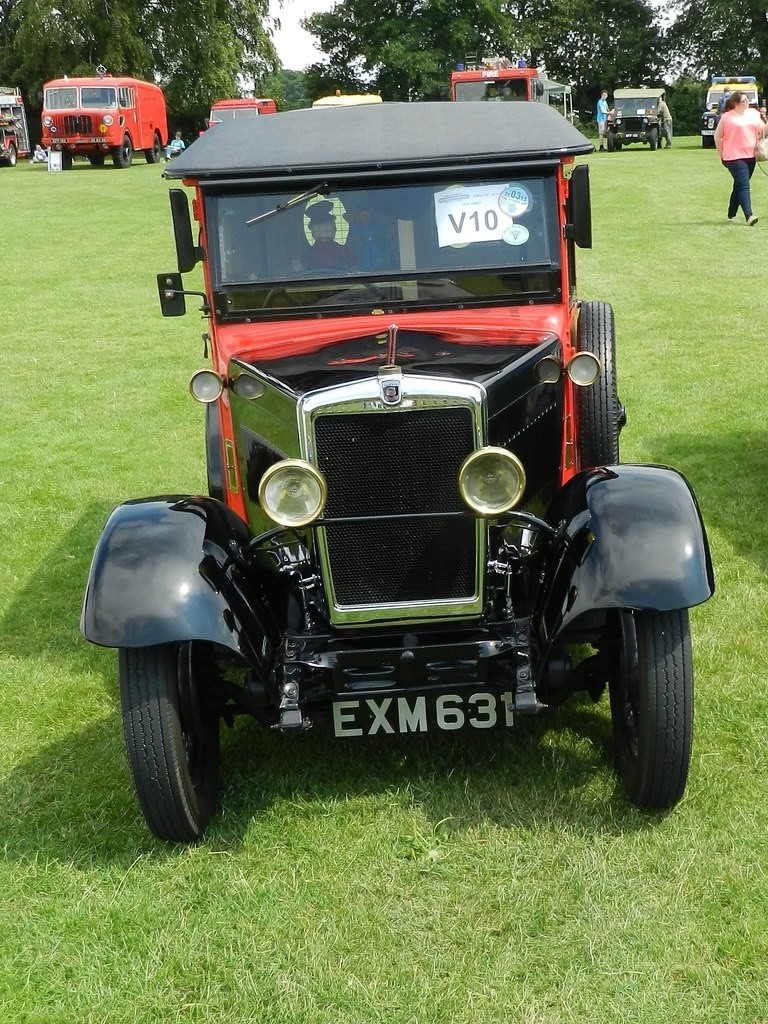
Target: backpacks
[721,92,733,112]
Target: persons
[597,90,624,151]
[287,200,389,271]
[163,131,185,163]
[32,145,46,163]
[714,87,768,226]
[658,96,673,149]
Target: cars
[312,88,383,108]
[197,99,278,136]
[80,99,718,845]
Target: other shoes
[727,217,739,224]
[664,144,671,149]
[599,148,608,151]
[748,215,758,226]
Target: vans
[41,64,168,169]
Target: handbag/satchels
[755,126,768,161]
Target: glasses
[739,97,747,104]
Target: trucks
[449,50,550,106]
[698,75,761,150]
[0,85,32,167]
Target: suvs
[604,87,668,153]
